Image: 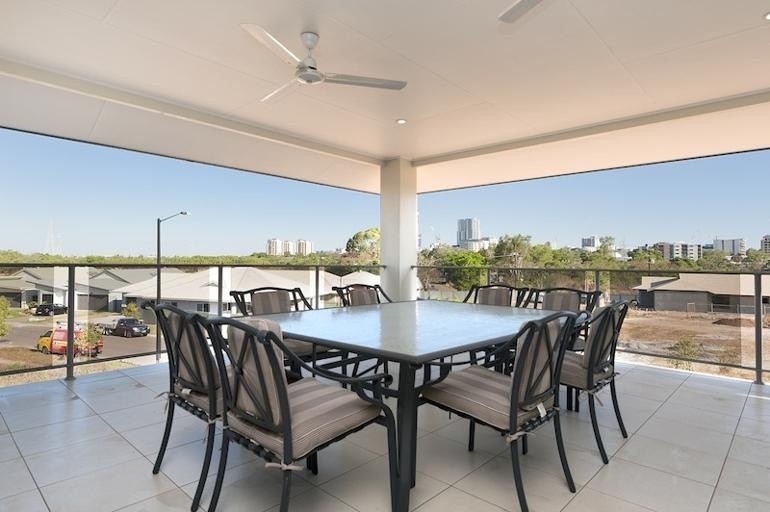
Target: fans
[239,23,407,105]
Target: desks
[229,298,587,512]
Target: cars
[36,303,68,315]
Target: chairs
[522,287,603,412]
[147,299,312,512]
[410,310,578,512]
[521,299,630,464]
[331,283,393,399]
[462,284,530,307]
[201,315,398,512]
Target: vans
[36,328,104,357]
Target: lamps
[395,118,409,126]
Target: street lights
[157,211,192,351]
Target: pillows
[229,286,349,389]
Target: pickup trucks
[102,318,150,338]
